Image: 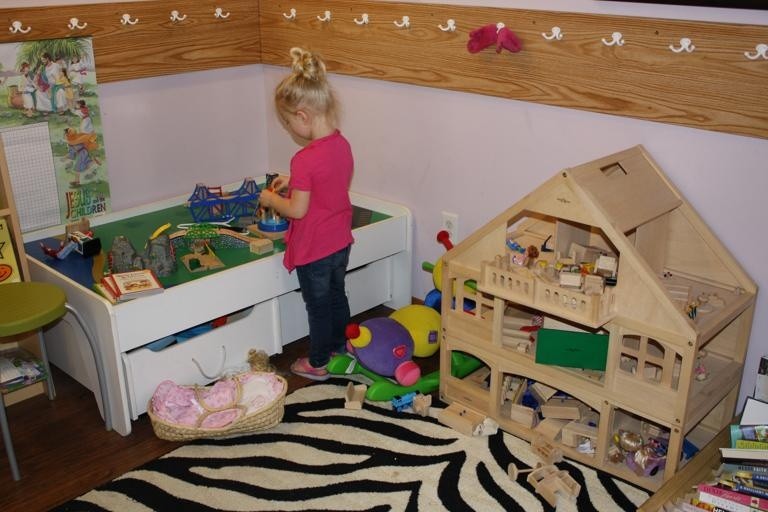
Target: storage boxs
[122,296,284,421]
[278,255,395,347]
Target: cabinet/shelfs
[0,138,55,410]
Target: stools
[0,282,111,482]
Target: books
[683,395,768,512]
[93,268,165,305]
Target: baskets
[146,369,289,443]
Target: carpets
[50,363,686,512]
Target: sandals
[289,357,330,383]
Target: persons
[259,47,354,382]
[18,53,103,185]
